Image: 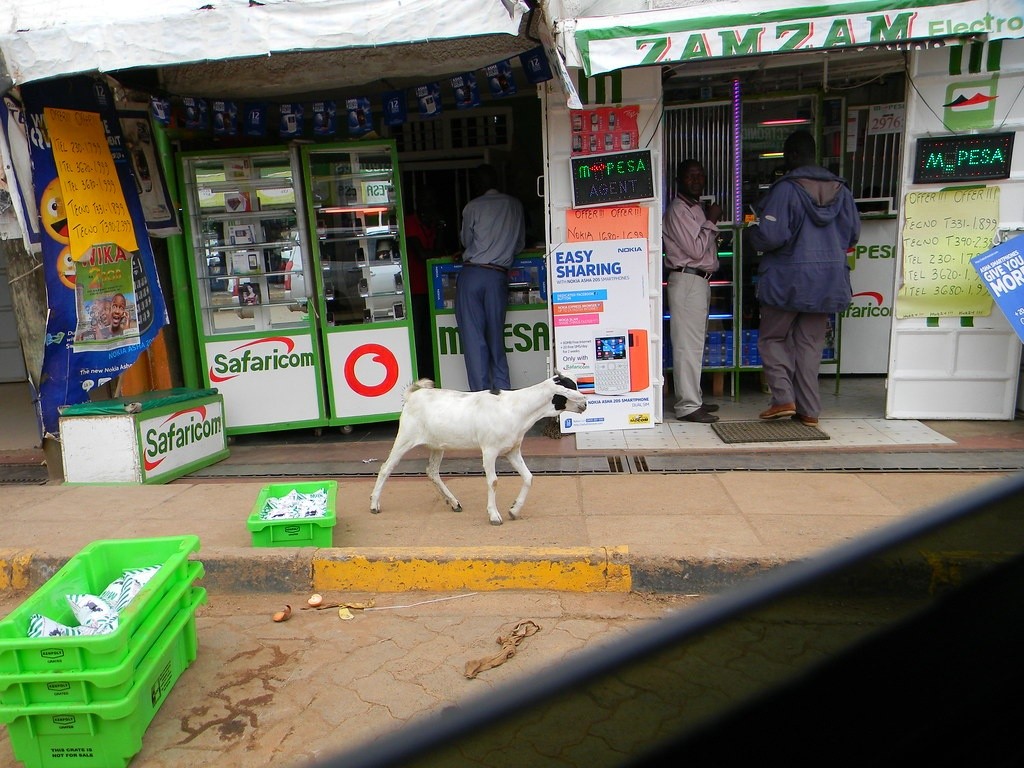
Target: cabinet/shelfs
[177,139,419,434]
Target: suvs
[283,225,406,323]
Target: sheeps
[370,366,587,526]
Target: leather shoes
[700,403,719,412]
[676,407,720,423]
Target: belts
[462,263,508,272]
[672,267,713,281]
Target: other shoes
[791,412,818,426]
[758,402,796,419]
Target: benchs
[57,387,230,485]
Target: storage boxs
[0,533,208,768]
[248,480,338,548]
[662,313,835,367]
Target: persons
[244,285,258,305]
[404,183,439,378]
[454,165,527,391]
[662,159,724,422]
[747,130,861,426]
[88,292,137,340]
